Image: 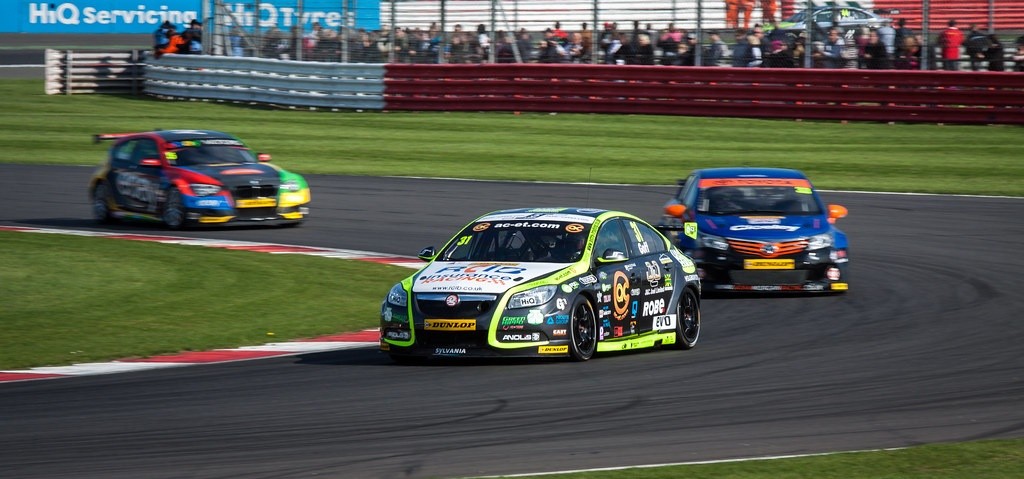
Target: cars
[379,207,702,363]
[88,127,311,229]
[761,4,900,41]
[661,166,850,295]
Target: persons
[537,235,586,261]
[156,0,1023,73]
